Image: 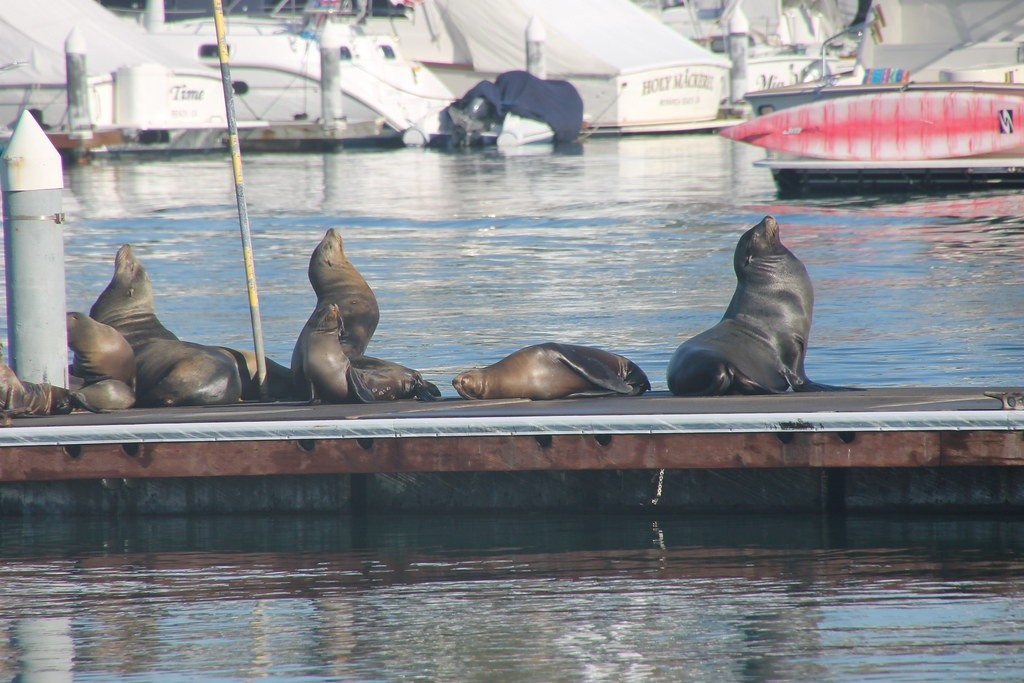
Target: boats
[0,1,1024,188]
